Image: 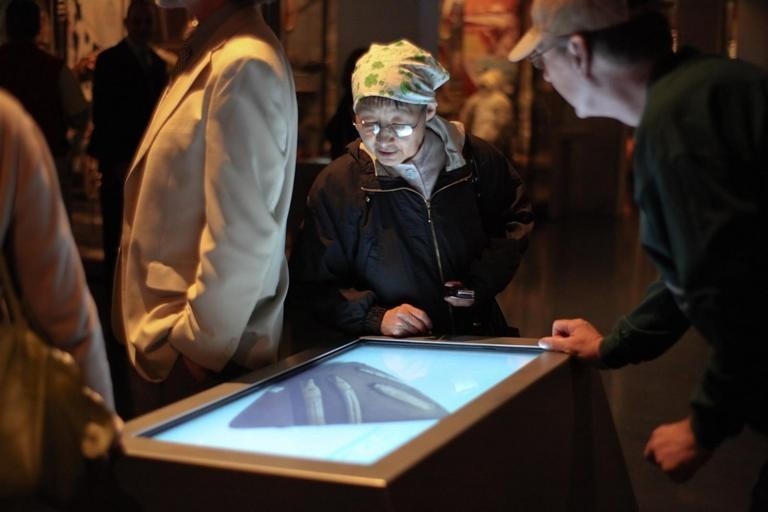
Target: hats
[507,0,675,64]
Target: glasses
[351,106,426,137]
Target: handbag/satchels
[0,246,127,511]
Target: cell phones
[445,284,477,300]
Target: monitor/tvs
[117,332,571,489]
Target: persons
[508,0,768,511]
[110,1,299,418]
[90,0,172,266]
[294,38,534,337]
[0,91,120,512]
[0,0,87,222]
